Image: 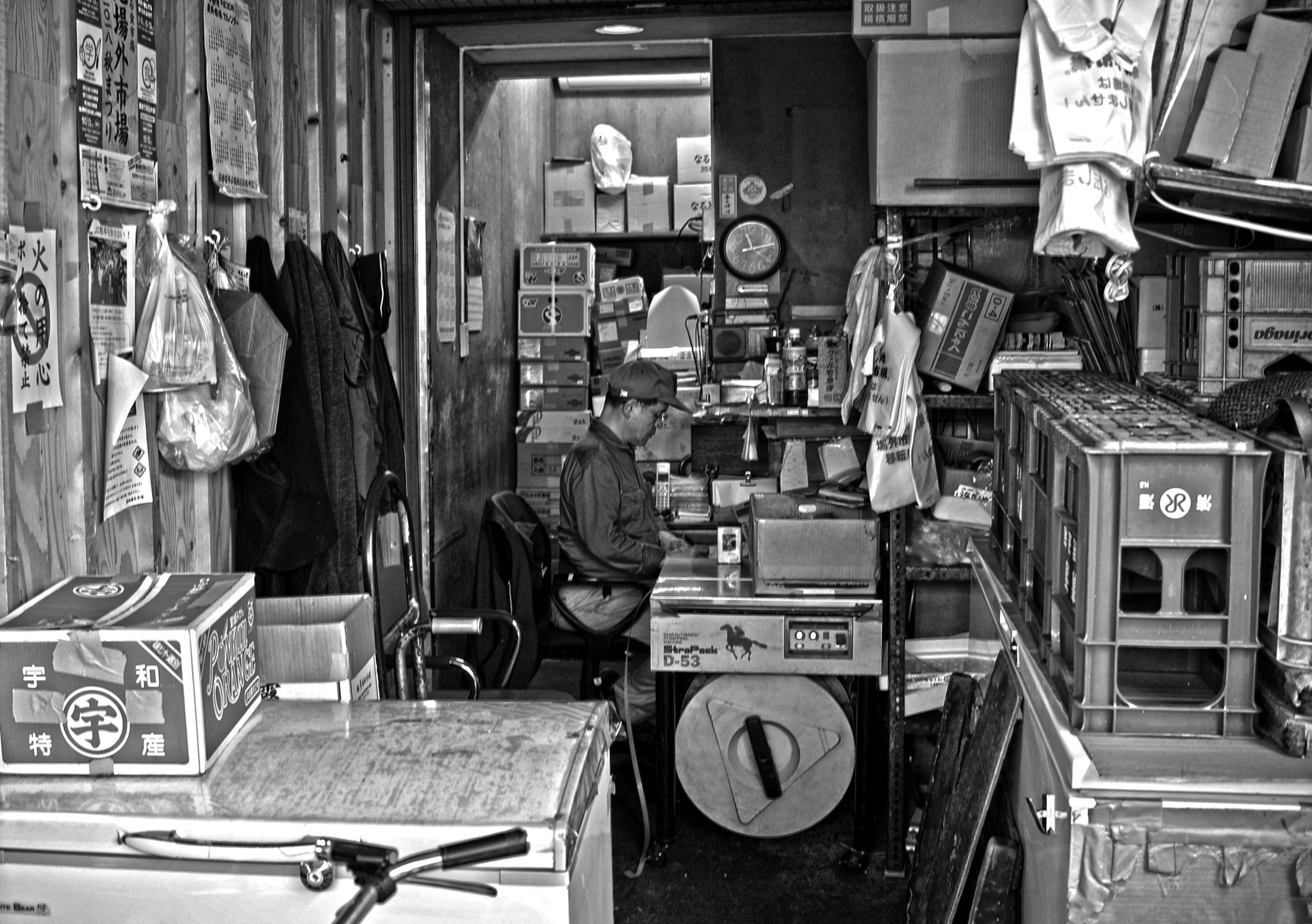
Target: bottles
[782,327,808,407]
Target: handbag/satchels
[134,224,218,392]
[156,286,259,475]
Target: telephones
[653,460,675,522]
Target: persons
[552,356,692,742]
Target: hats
[606,358,698,417]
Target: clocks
[719,214,785,282]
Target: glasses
[641,401,668,428]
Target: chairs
[462,489,652,702]
[361,472,577,702]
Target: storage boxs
[850,0,1027,62]
[866,40,1043,207]
[932,434,993,527]
[514,241,691,590]
[748,491,882,595]
[544,137,716,243]
[1128,273,1166,350]
[914,258,1015,394]
[0,572,262,776]
[256,594,381,701]
[987,367,1274,740]
[1173,14,1312,181]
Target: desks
[645,549,885,871]
